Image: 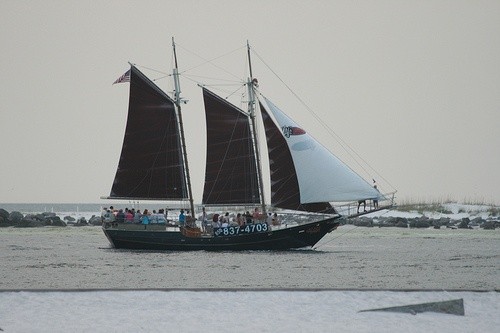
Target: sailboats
[94,35,400,252]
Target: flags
[112,69,130,85]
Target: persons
[201,207,207,235]
[103,206,167,225]
[186,211,191,227]
[242,207,281,226]
[179,209,185,226]
[373,185,379,209]
[357,200,367,214]
[211,212,244,228]
